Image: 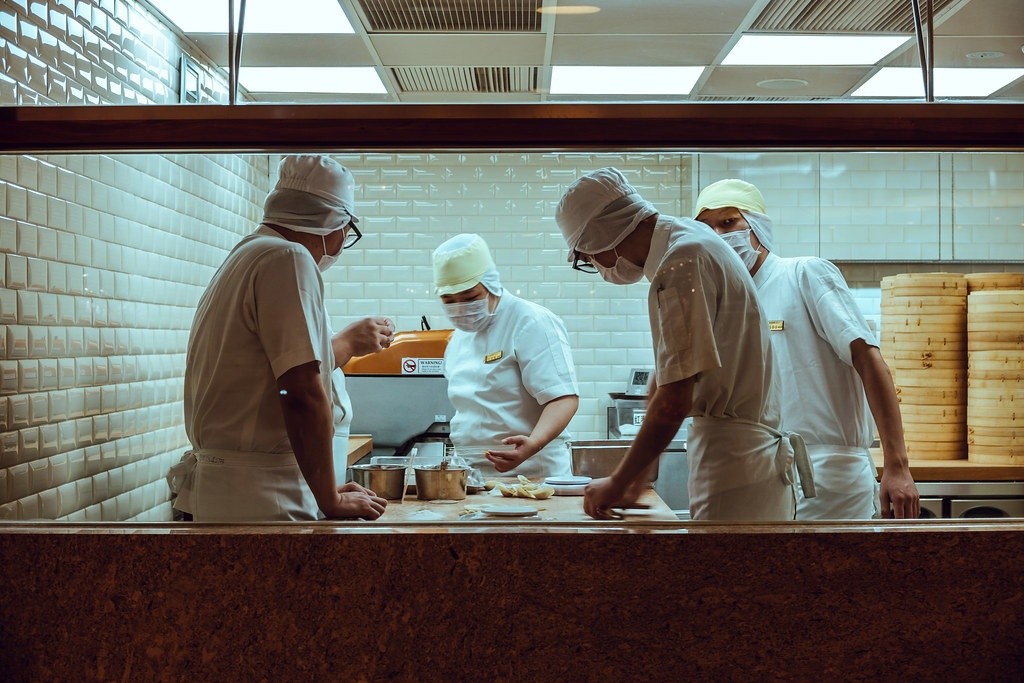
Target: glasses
[710,220,747,235]
[342,221,363,253]
[571,252,599,274]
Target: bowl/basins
[347,464,407,502]
[412,464,469,500]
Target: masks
[440,291,497,332]
[591,248,644,285]
[317,228,346,272]
[718,227,762,272]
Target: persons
[163,155,395,523]
[692,178,920,519]
[431,233,579,478]
[554,166,817,519]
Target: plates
[543,476,592,484]
[481,509,538,516]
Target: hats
[432,233,492,296]
[275,154,359,224]
[693,179,767,220]
[554,167,637,262]
